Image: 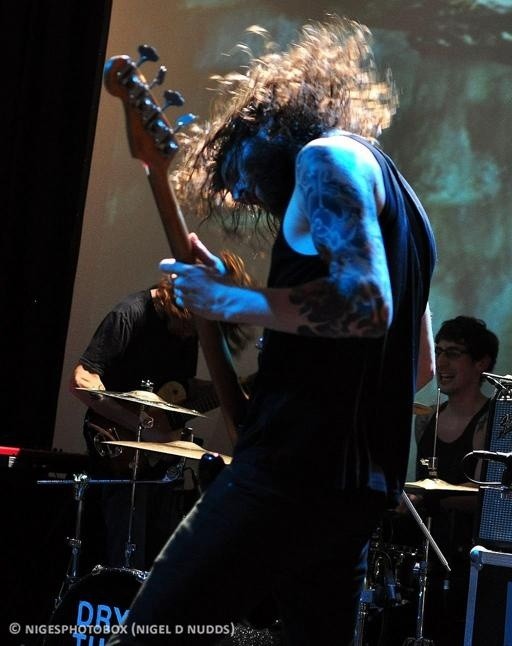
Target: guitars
[102,44,261,500]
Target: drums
[374,542,451,599]
[41,564,149,646]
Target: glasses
[435,346,468,360]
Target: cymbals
[75,386,209,421]
[101,438,233,467]
[403,477,479,498]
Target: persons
[102,8,438,646]
[71,249,262,572]
[394,314,500,518]
